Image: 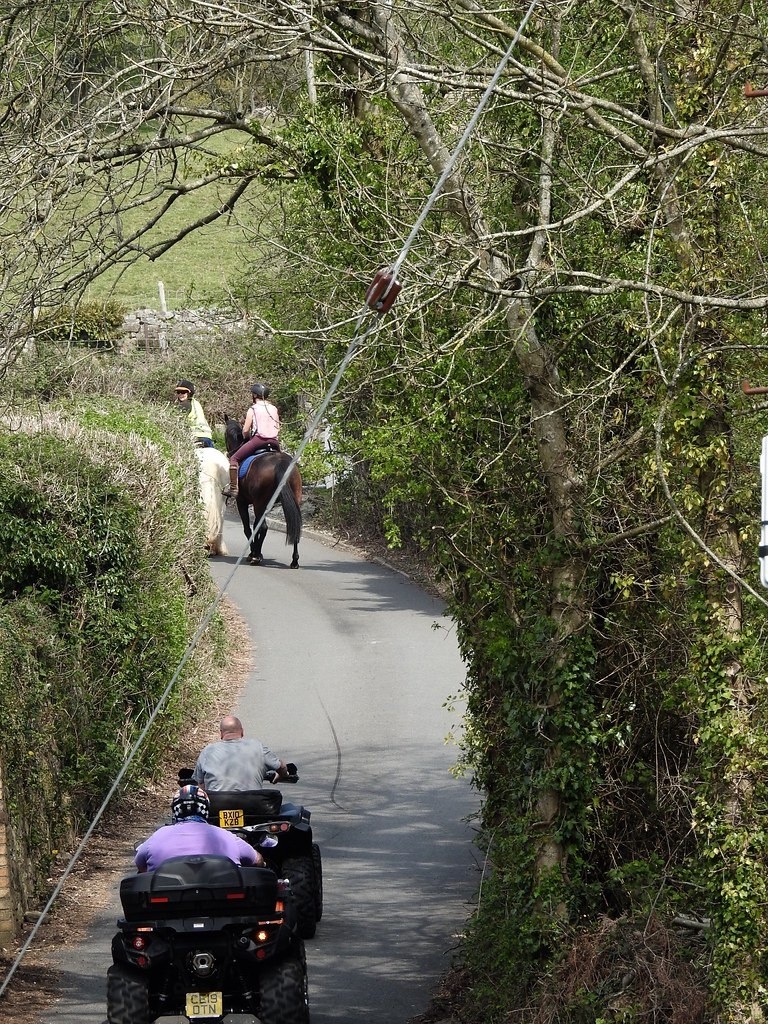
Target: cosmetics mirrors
[259,832,279,848]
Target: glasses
[176,391,186,394]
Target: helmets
[174,381,196,392]
[251,382,270,398]
[171,786,211,806]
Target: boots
[223,467,239,498]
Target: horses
[224,413,302,569]
[193,447,232,559]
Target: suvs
[176,763,323,940]
[104,838,313,1024]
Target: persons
[131,783,268,876]
[172,380,215,449]
[190,715,290,792]
[221,383,281,498]
